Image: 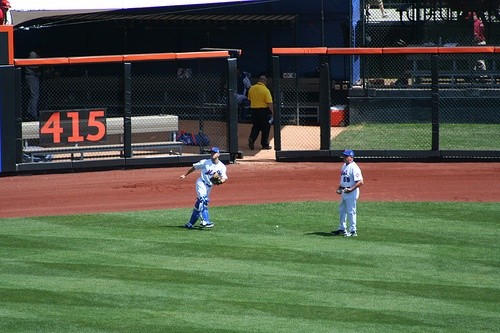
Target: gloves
[180,175,185,179]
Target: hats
[341,149,355,157]
[211,147,220,153]
[466,11,476,19]
[0,0,11,8]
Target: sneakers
[343,230,357,237]
[199,220,214,228]
[331,230,348,236]
[186,223,192,228]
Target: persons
[248,74,274,149]
[236,65,252,120]
[179,147,228,229]
[391,78,408,86]
[334,149,364,237]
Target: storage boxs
[330,105,349,127]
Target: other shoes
[262,146,272,149]
[248,138,255,151]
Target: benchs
[405,42,500,89]
[22,115,183,163]
